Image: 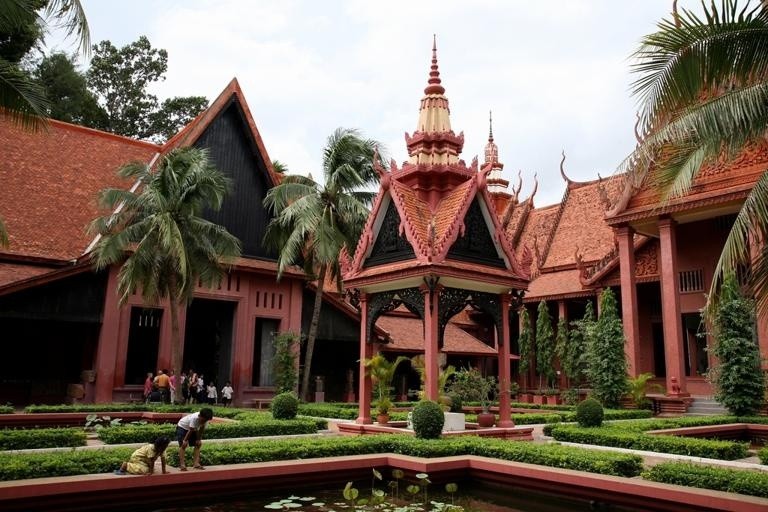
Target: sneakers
[113,471,125,474]
[181,465,187,470]
[194,465,205,470]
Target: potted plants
[370,396,394,423]
[472,388,513,426]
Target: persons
[176,408,212,471]
[143,369,234,407]
[120,436,171,474]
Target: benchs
[252,398,272,409]
